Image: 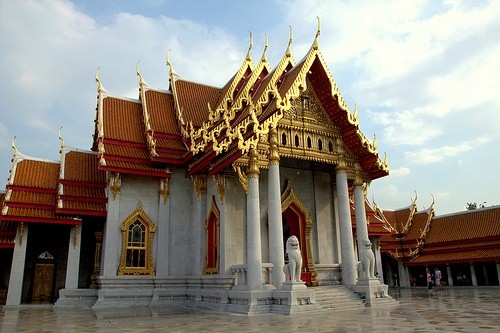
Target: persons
[393,272,399,287]
[410,275,416,287]
[427,269,432,292]
[456,272,468,286]
[435,268,443,291]
